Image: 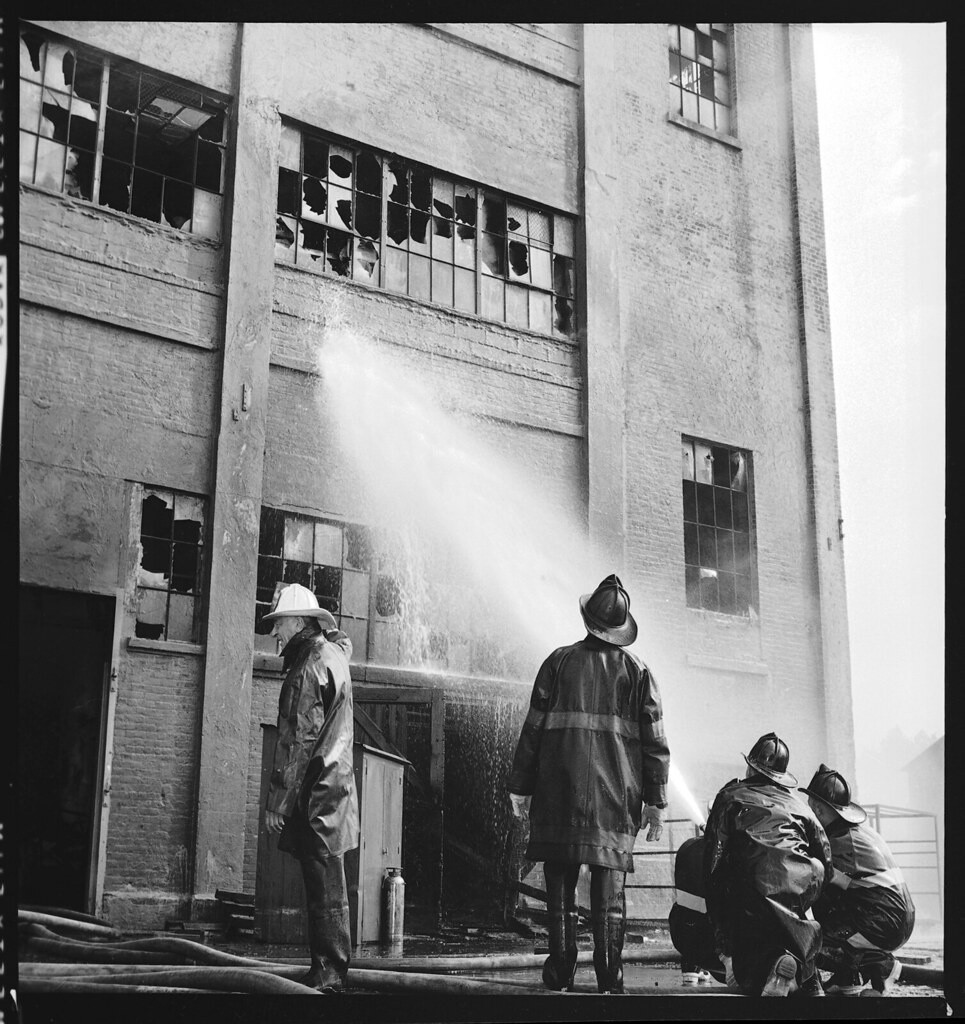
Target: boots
[593,912,627,996]
[543,910,578,992]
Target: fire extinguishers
[382,866,407,959]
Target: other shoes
[681,962,711,982]
[860,954,903,997]
[760,954,797,997]
[819,971,862,994]
[801,977,825,997]
[719,951,743,992]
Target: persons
[508,574,670,993]
[667,731,916,997]
[262,584,360,990]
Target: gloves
[509,792,532,823]
[640,802,666,842]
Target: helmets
[579,574,638,647]
[741,732,798,788]
[263,583,338,630]
[797,763,867,823]
